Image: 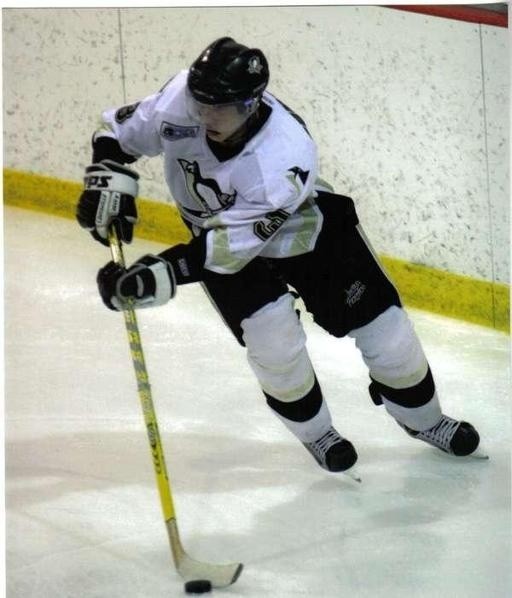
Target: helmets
[188,35,271,103]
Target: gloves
[96,254,177,311]
[76,160,140,246]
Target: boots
[294,427,358,472]
[404,415,480,456]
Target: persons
[74,35,481,474]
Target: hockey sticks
[108,223,244,586]
